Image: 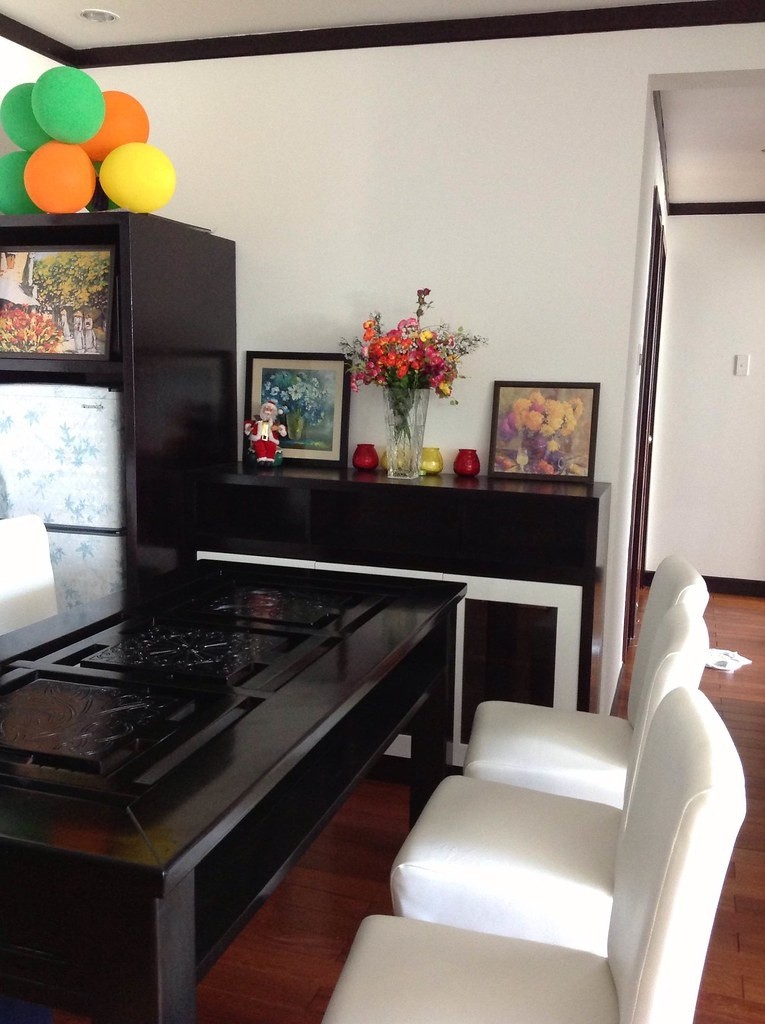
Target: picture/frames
[488,380,600,486]
[0,245,116,361]
[241,349,352,471]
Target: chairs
[463,555,710,802]
[0,514,58,636]
[318,685,745,1023]
[389,601,708,955]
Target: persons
[244,401,287,471]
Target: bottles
[453,449,481,477]
[352,444,379,471]
[420,447,443,475]
[381,449,403,471]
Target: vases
[286,413,304,440]
[382,385,431,480]
[526,436,548,462]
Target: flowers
[262,370,327,427]
[500,390,583,451]
[336,287,489,408]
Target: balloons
[32,67,106,144]
[79,91,149,162]
[0,149,45,215]
[0,82,54,152]
[99,143,176,213]
[25,140,96,214]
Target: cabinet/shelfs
[0,209,240,612]
[200,460,611,789]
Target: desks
[0,558,466,1024]
